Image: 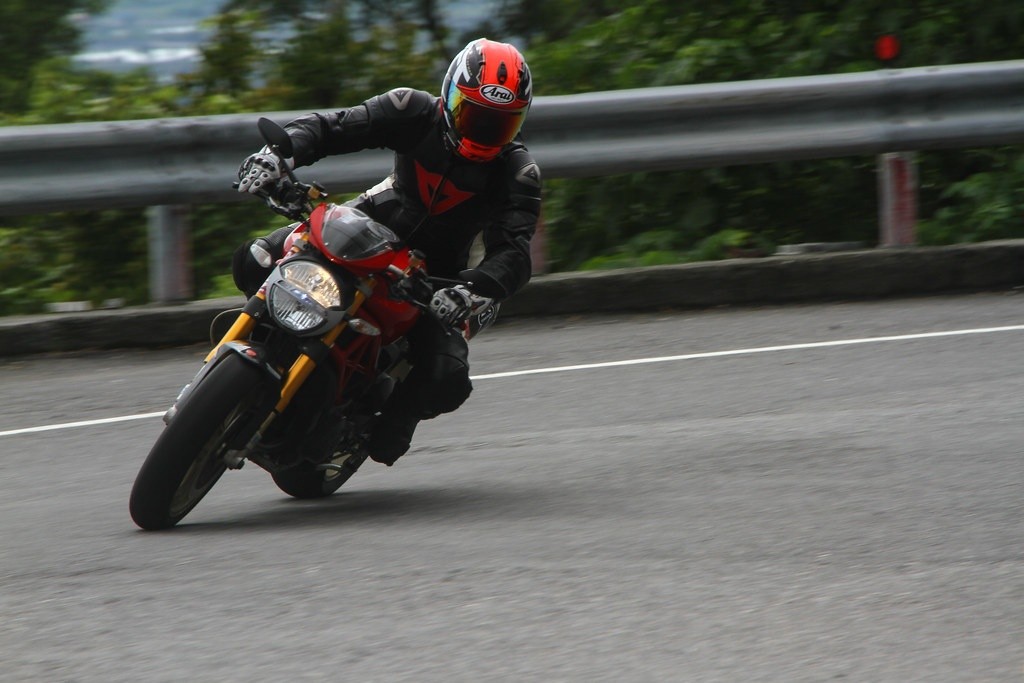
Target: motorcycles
[128,117,507,532]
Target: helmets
[440,37,534,164]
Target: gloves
[236,145,296,193]
[430,284,494,328]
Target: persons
[230,38,540,467]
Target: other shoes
[368,383,419,466]
